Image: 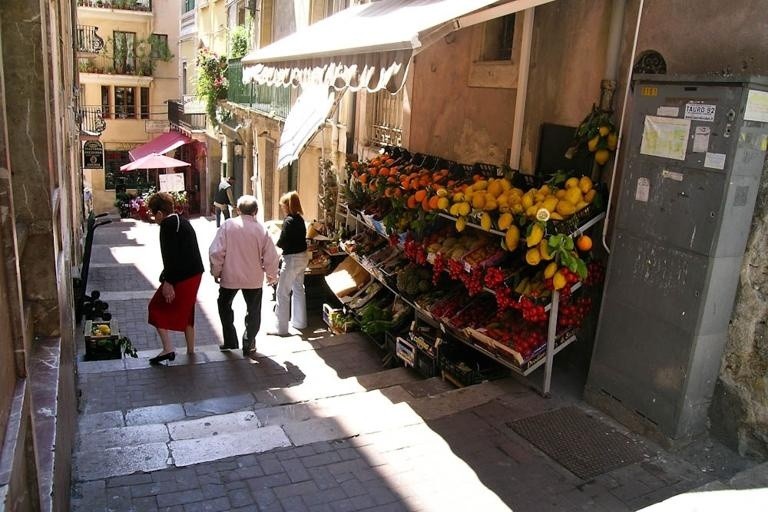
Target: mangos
[450,177,593,290]
[588,127,617,163]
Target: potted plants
[87,63,150,76]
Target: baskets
[437,342,507,386]
[383,145,609,239]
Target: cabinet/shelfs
[336,144,609,399]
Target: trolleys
[70,210,115,323]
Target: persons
[144,192,204,364]
[214,176,238,228]
[266,190,309,337]
[208,194,280,356]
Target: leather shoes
[243,348,256,356]
[219,344,239,349]
[149,351,175,362]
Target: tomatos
[432,255,601,356]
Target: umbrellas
[119,150,192,191]
